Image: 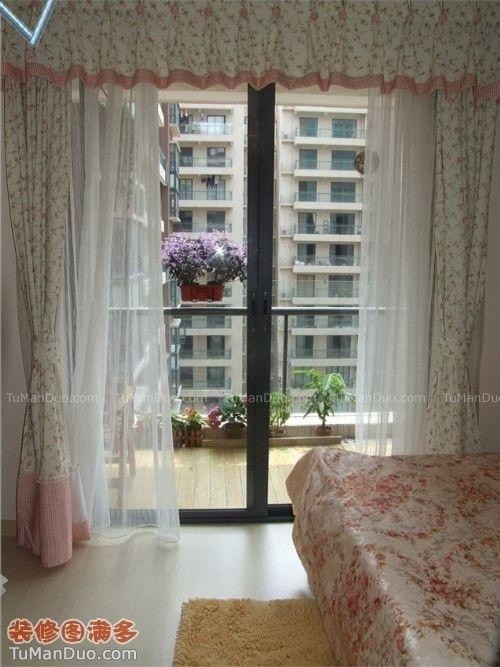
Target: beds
[286,445,499,665]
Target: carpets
[172,598,337,666]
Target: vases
[184,429,203,446]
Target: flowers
[181,407,206,430]
[203,406,221,429]
[161,229,249,287]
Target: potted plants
[181,284,223,301]
[268,392,292,438]
[291,368,355,436]
[220,396,247,439]
